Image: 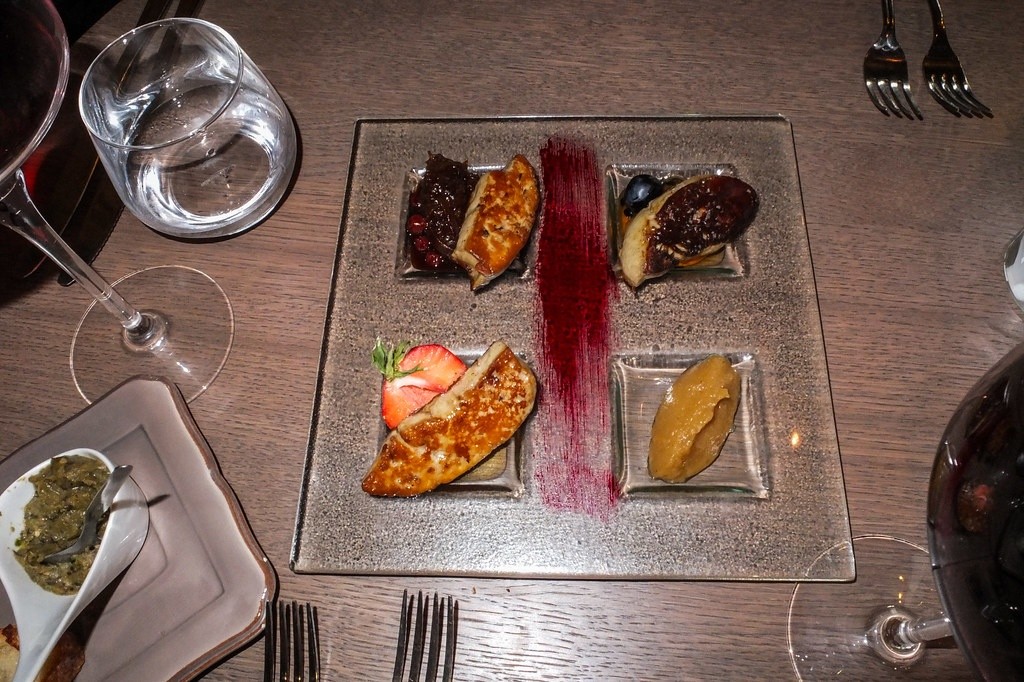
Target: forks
[392,588,455,682]
[264,600,320,682]
[863,0,922,114]
[923,0,993,114]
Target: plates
[285,112,857,582]
[0,375,277,682]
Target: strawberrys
[371,337,467,430]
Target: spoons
[41,464,133,565]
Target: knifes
[57,0,206,286]
[16,0,172,280]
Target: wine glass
[786,341,1024,682]
[1,0,234,405]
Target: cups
[78,16,297,239]
